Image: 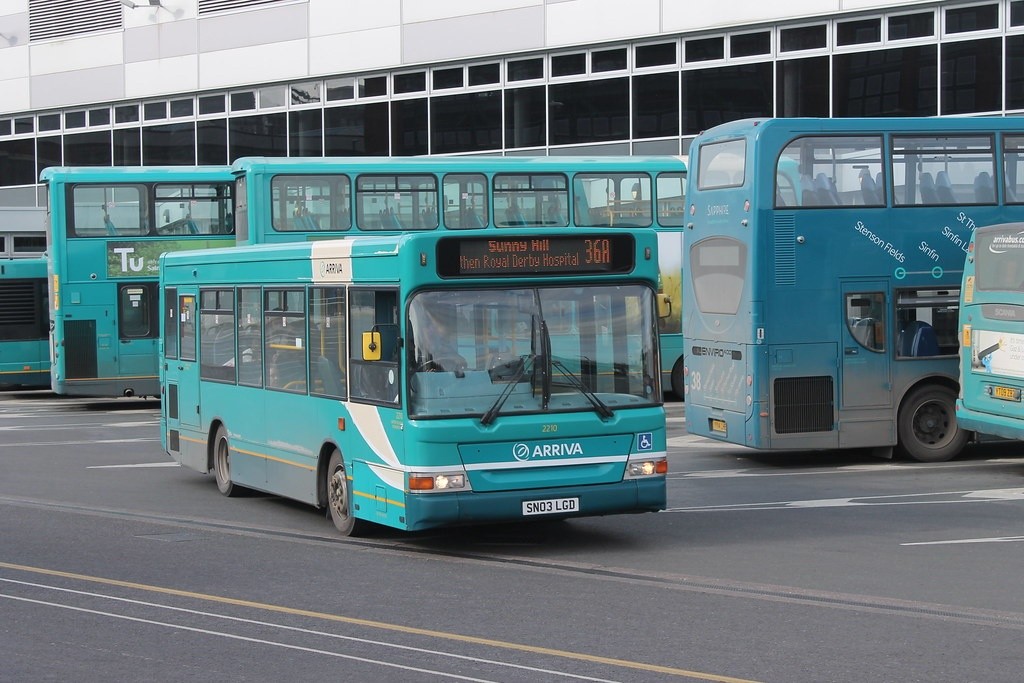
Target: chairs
[775,182,787,206]
[829,177,843,205]
[102,205,567,236]
[876,173,899,204]
[990,173,1018,202]
[816,173,838,205]
[920,173,941,202]
[182,318,344,396]
[800,174,819,206]
[974,172,994,202]
[936,171,960,202]
[859,167,880,204]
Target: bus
[682,110,1024,463]
[40,156,801,536]
[0,258,52,392]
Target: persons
[404,317,466,372]
